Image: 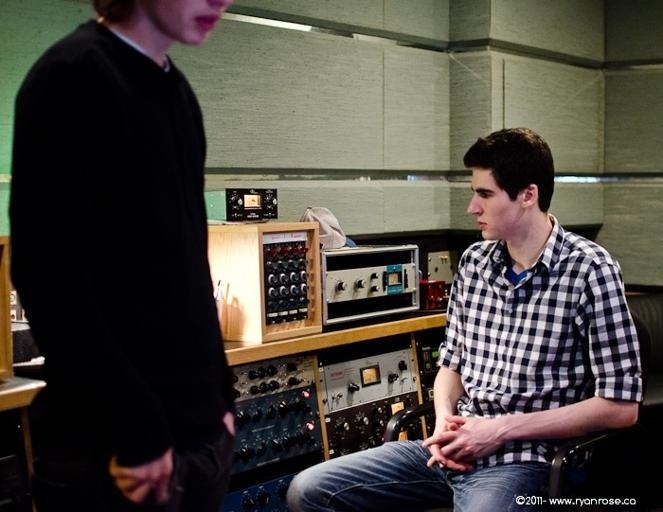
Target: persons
[8,1,240,511]
[285,127,643,512]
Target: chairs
[378,308,652,512]
